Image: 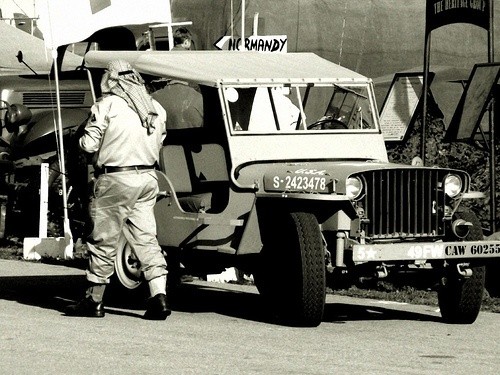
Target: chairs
[160,140,235,213]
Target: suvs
[0,17,103,244]
[64,43,489,328]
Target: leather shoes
[142,292,171,320]
[64,295,105,317]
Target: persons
[62,60,174,321]
[170,27,194,51]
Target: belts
[99,165,154,174]
